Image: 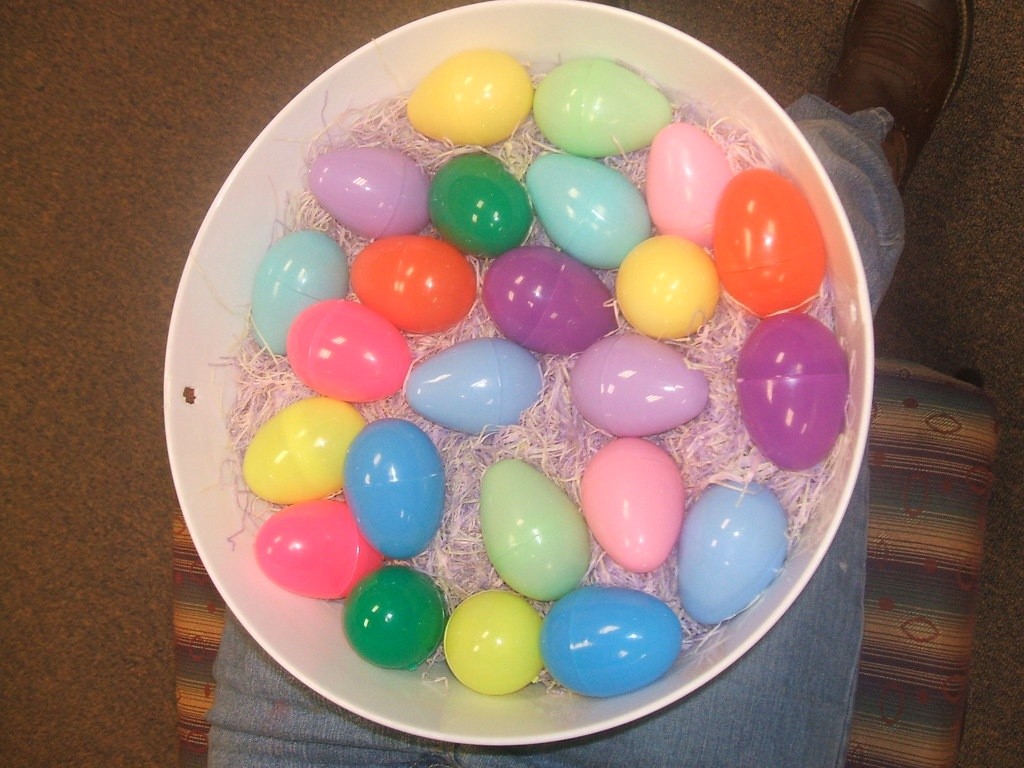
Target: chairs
[171,351,1001,768]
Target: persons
[207,0,975,768]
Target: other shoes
[824,0,975,197]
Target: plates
[163,1,874,745]
